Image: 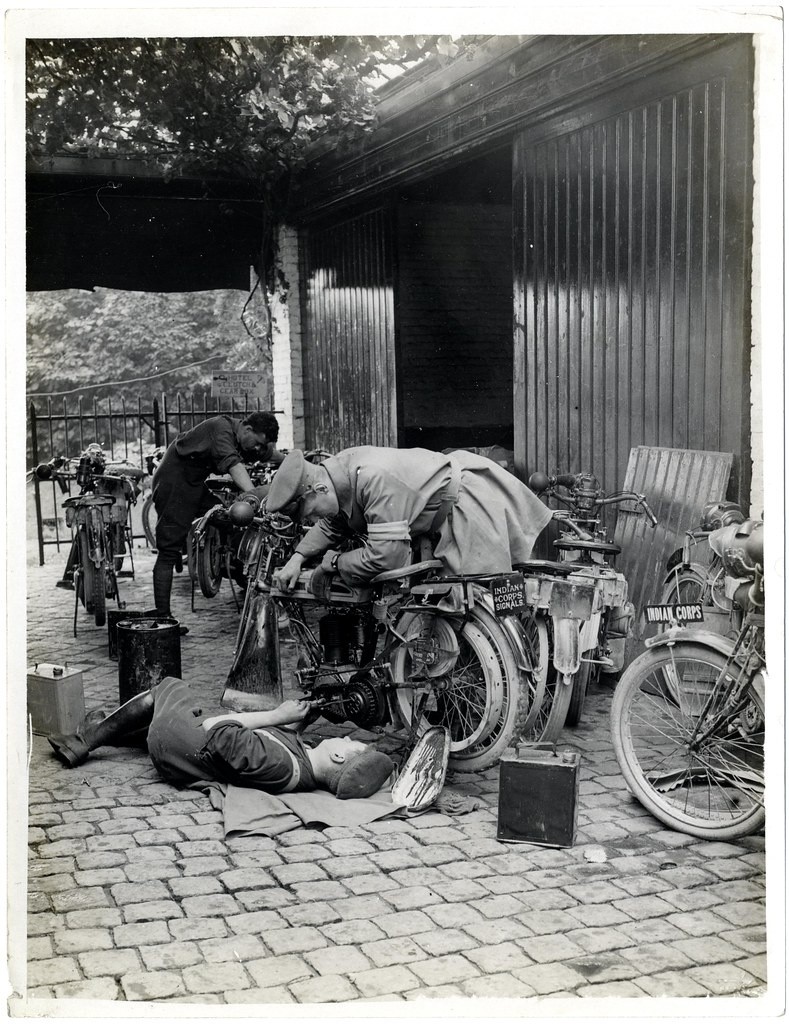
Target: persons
[45,676,392,801]
[152,412,288,634]
[265,446,552,595]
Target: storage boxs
[683,605,764,682]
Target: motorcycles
[609,498,765,839]
[142,455,661,776]
[26,444,147,631]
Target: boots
[47,689,155,768]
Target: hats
[330,745,394,799]
[265,448,316,524]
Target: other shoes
[179,627,189,636]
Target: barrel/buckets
[116,618,182,706]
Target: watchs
[330,554,341,570]
